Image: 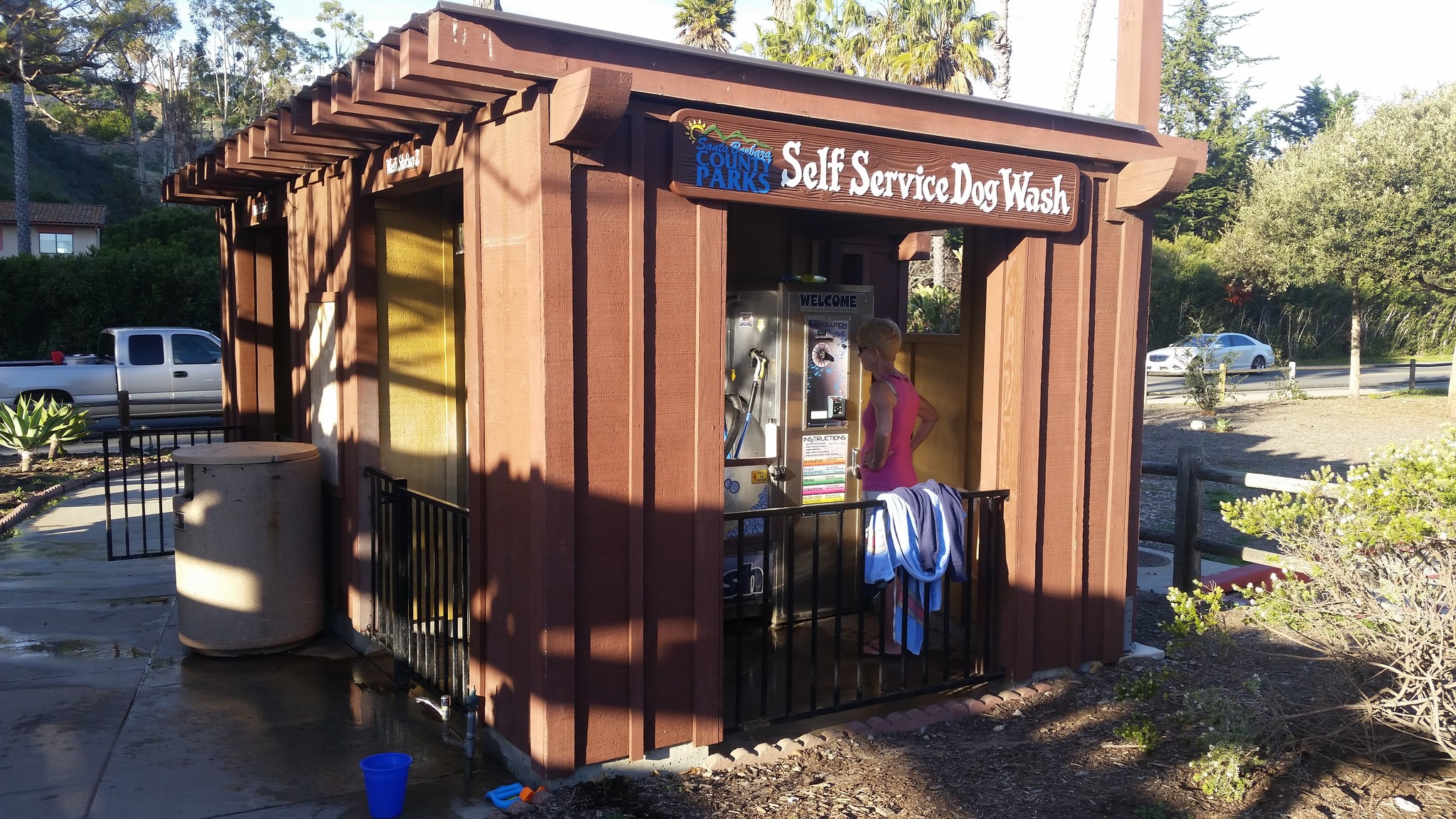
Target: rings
[864,458,867,463]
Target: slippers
[860,644,902,659]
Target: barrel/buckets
[360,752,413,819]
[51,351,63,364]
[62,354,98,365]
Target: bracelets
[872,458,882,466]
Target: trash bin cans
[165,438,332,657]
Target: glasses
[856,345,867,355]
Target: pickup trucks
[0,319,223,447]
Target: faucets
[415,695,451,721]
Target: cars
[1146,332,1276,378]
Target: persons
[857,318,939,662]
